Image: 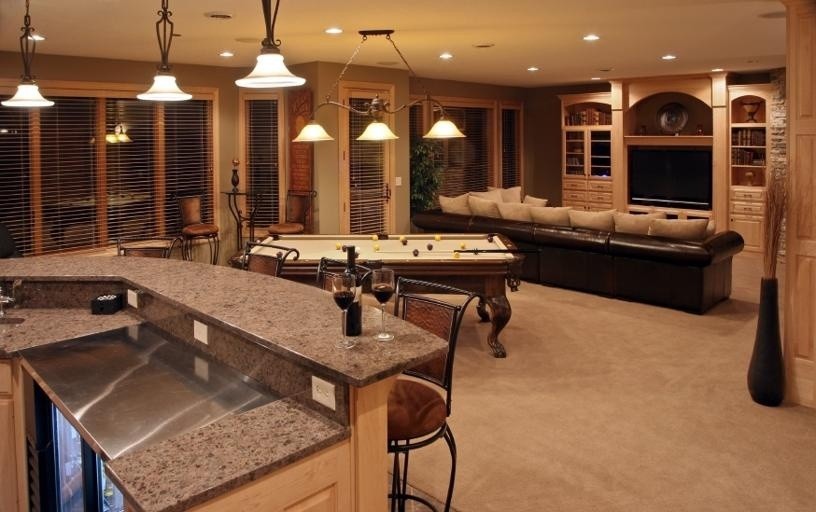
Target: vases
[231,170,239,191]
[747,277,785,407]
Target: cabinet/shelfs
[556,74,773,250]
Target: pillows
[438,186,716,242]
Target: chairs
[242,242,299,277]
[116,236,184,259]
[172,193,219,265]
[267,188,317,234]
[387,277,484,512]
[317,257,372,299]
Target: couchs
[533,224,745,316]
[413,208,539,287]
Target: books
[565,108,612,175]
[730,127,766,165]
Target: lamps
[0,0,57,108]
[291,30,467,143]
[137,0,192,102]
[235,0,307,88]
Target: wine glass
[370,268,395,343]
[743,102,761,123]
[330,272,355,350]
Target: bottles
[341,245,362,338]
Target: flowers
[232,158,240,170]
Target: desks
[231,232,522,358]
[220,191,271,251]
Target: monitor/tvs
[626,145,714,209]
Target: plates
[658,103,690,133]
